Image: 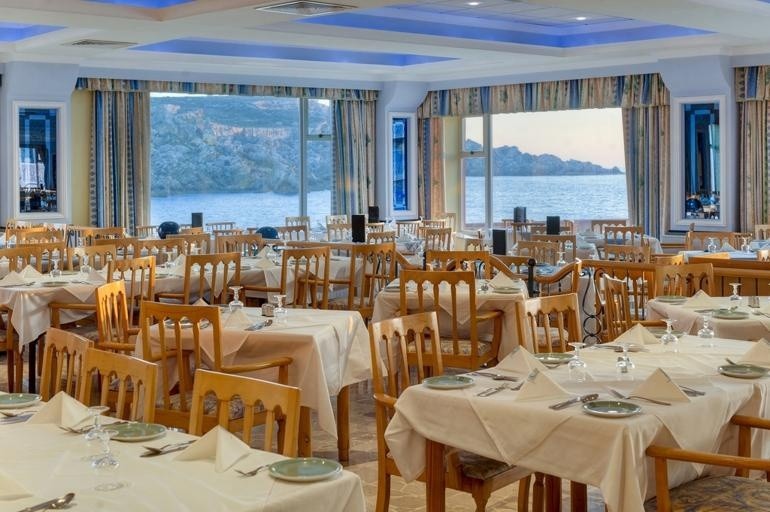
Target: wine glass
[36,242,317,285]
[660,319,682,355]
[614,341,638,382]
[85,403,110,455]
[697,311,717,346]
[705,232,751,257]
[91,427,123,491]
[567,342,587,384]
[227,286,288,332]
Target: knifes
[16,499,50,510]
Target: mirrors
[11,99,66,219]
[387,111,417,218]
[672,95,727,228]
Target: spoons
[43,490,75,511]
[549,393,600,411]
[139,434,198,457]
[2,410,39,417]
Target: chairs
[79,347,162,431]
[187,366,304,456]
[135,300,292,447]
[32,324,97,410]
[372,218,770,397]
[603,415,770,512]
[365,311,536,512]
[0,217,454,393]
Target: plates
[213,304,229,313]
[421,372,474,392]
[268,456,343,483]
[0,391,42,410]
[717,364,766,379]
[583,401,640,416]
[164,317,208,329]
[530,353,573,364]
[112,422,167,442]
[382,284,408,294]
[494,284,523,295]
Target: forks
[234,463,272,478]
[57,413,128,434]
[474,371,524,399]
[610,389,670,404]
[726,358,770,369]
